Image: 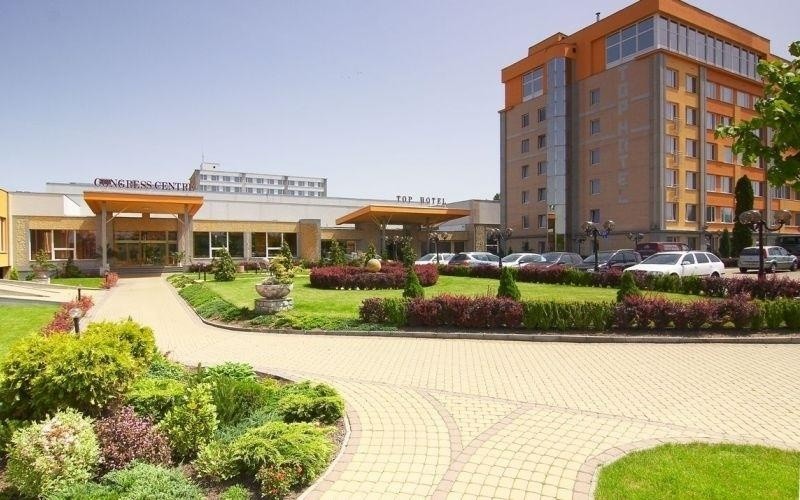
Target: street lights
[581,220,616,274]
[428,232,453,264]
[486,228,514,267]
[624,232,644,246]
[738,209,792,280]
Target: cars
[738,245,798,273]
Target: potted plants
[254,256,295,300]
